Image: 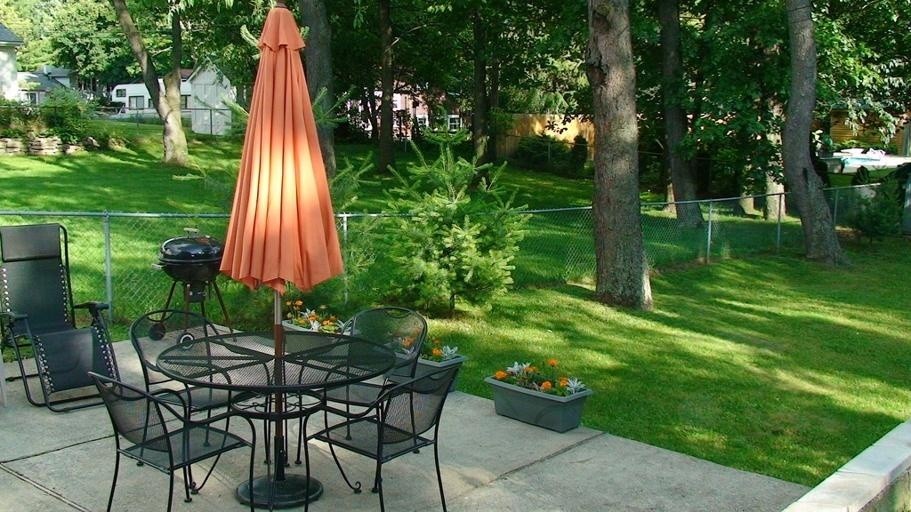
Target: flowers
[286,299,343,332]
[495,354,585,394]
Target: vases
[485,374,593,433]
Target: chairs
[1,222,123,414]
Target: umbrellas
[219,0,345,437]
